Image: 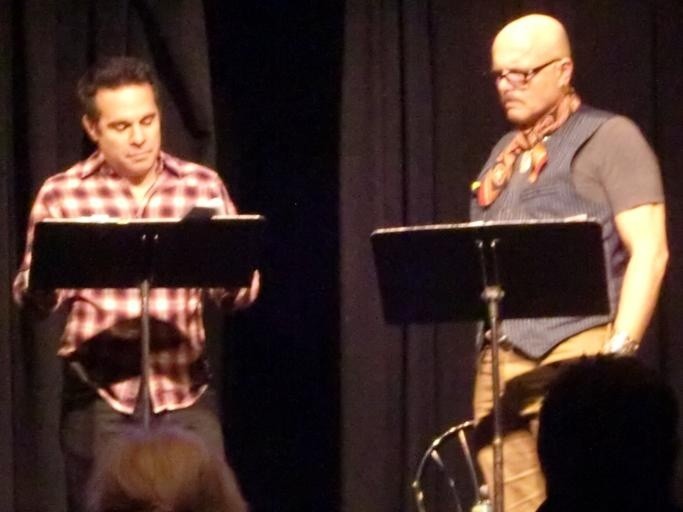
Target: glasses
[491,58,557,85]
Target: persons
[13,55,261,512]
[469,12,671,503]
[85,424,243,511]
[536,351,683,511]
